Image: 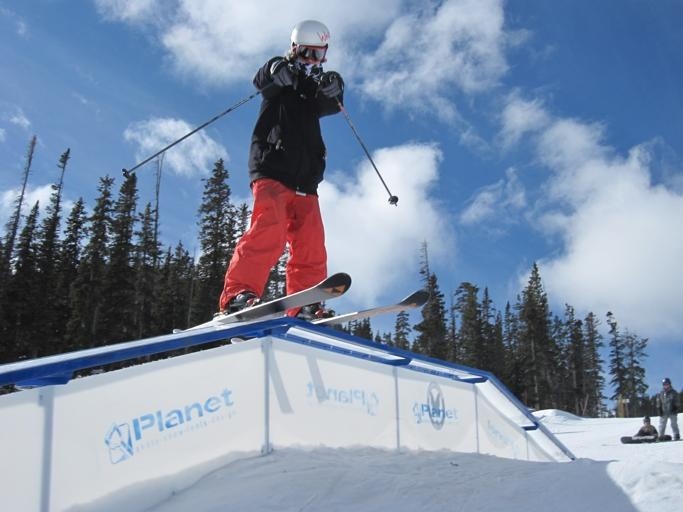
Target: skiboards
[172,273,430,343]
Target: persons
[219,19,344,321]
[655,378,681,441]
[633,415,658,438]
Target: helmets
[662,377,671,385]
[290,20,330,54]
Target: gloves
[317,71,344,99]
[269,56,298,90]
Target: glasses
[296,45,326,59]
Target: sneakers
[225,291,262,316]
[242,300,243,301]
[302,302,336,322]
